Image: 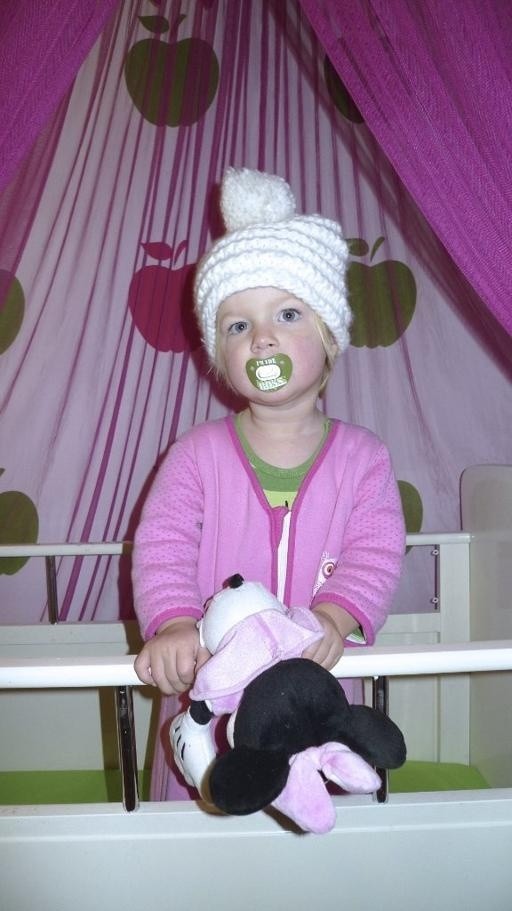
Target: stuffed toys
[157,570,408,837]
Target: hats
[191,211,353,361]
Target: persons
[118,228,413,806]
